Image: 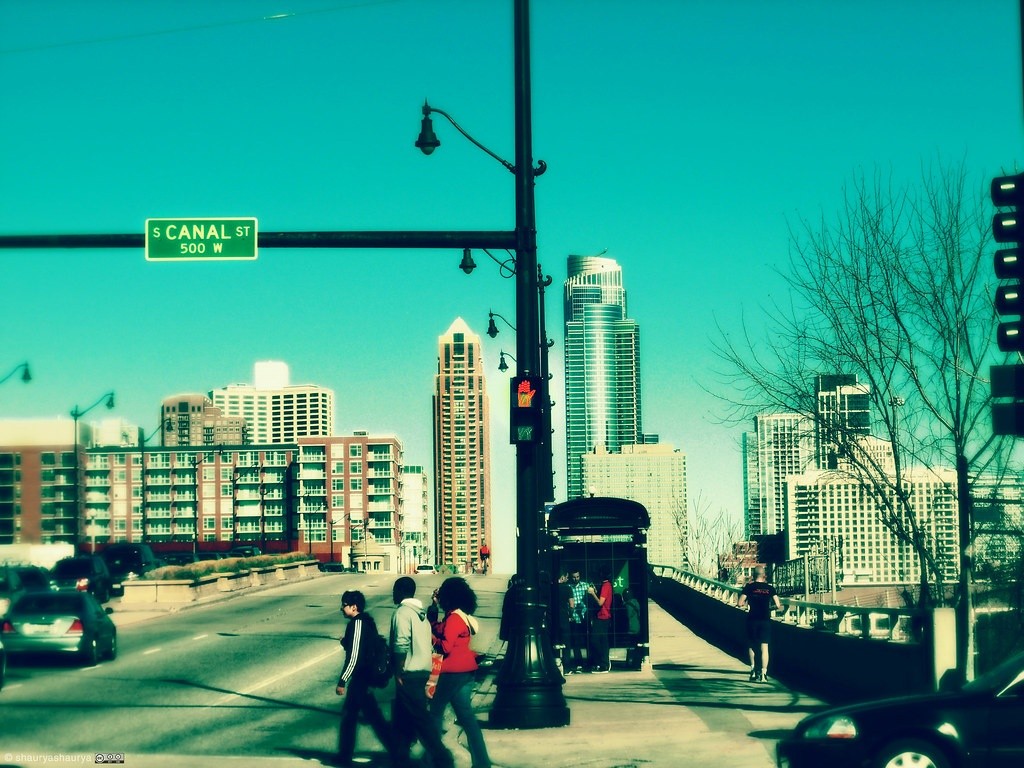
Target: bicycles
[482,556,489,575]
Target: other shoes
[575,666,584,673]
[591,668,609,674]
[564,670,575,676]
[750,671,768,683]
[630,665,641,670]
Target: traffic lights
[991,173,1023,440]
[507,375,544,445]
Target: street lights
[73,390,115,547]
[329,513,350,563]
[258,472,290,557]
[0,362,33,385]
[348,521,362,572]
[415,99,570,731]
[193,444,224,543]
[364,517,375,571]
[139,417,173,542]
[230,463,259,548]
[308,504,327,554]
[889,396,905,582]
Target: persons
[738,566,781,682]
[335,590,391,768]
[480,544,490,568]
[550,571,574,675]
[587,572,613,673]
[386,576,452,768]
[426,576,493,766]
[570,570,595,673]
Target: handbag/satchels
[362,637,397,689]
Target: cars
[414,564,438,575]
[98,542,263,586]
[322,563,345,573]
[0,590,115,666]
[775,649,1024,768]
[50,554,138,604]
[0,563,51,617]
